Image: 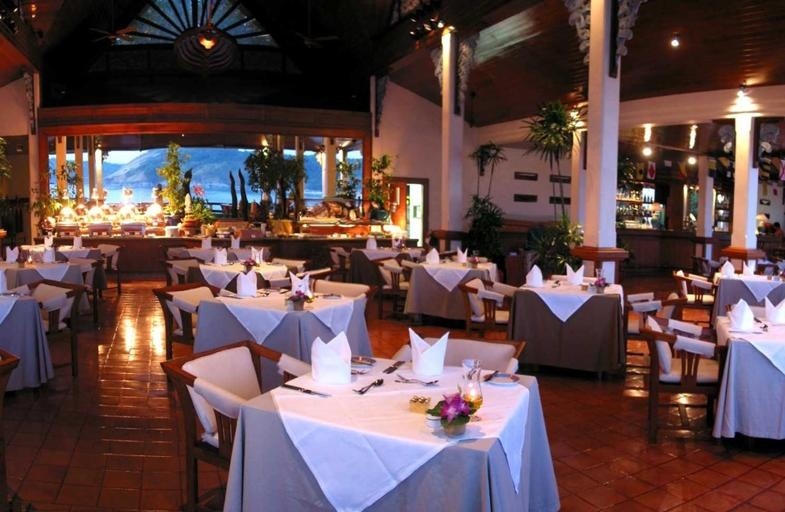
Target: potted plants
[242,145,308,214]
[362,154,399,220]
[31,187,62,234]
[335,159,361,210]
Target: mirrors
[383,176,428,248]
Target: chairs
[672,270,719,306]
[4,278,87,377]
[88,224,113,236]
[81,259,105,325]
[98,244,122,298]
[325,244,354,282]
[691,256,718,278]
[638,314,731,444]
[392,337,525,374]
[159,338,311,511]
[159,256,205,287]
[121,225,145,237]
[457,276,517,341]
[624,291,687,377]
[373,256,417,321]
[151,283,226,361]
[54,226,79,235]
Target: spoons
[358,379,384,394]
[397,373,436,386]
[393,379,440,386]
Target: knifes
[383,360,405,373]
[282,382,331,398]
[484,370,498,382]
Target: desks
[616,228,694,277]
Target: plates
[484,373,518,385]
[352,365,371,373]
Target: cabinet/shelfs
[618,178,656,228]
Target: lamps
[670,32,681,50]
[110,0,279,80]
[724,79,765,118]
